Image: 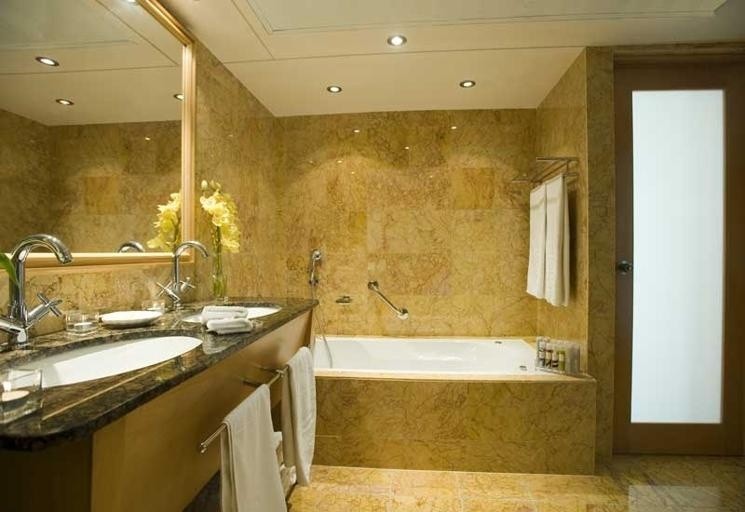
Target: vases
[209,253,231,300]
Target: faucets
[118,240,146,252]
[8,233,72,322]
[173,242,209,307]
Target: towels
[202,304,255,336]
[220,346,319,510]
[525,172,572,309]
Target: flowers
[147,190,182,253]
[198,180,244,286]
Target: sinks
[0,329,204,388]
[180,302,280,323]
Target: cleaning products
[537,338,579,375]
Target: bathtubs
[313,337,536,376]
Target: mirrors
[0,0,201,273]
